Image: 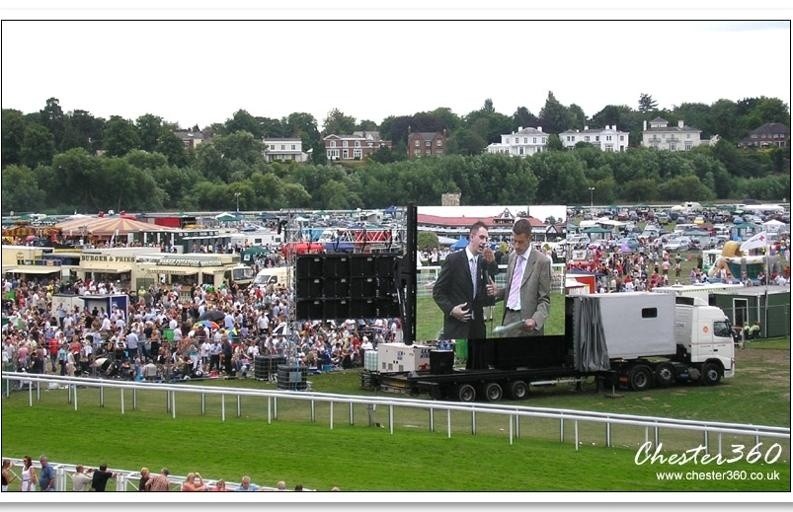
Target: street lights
[588,187,595,206]
[234,192,242,213]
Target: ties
[470,258,477,297]
[507,256,522,308]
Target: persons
[1,220,790,390]
[2,455,341,492]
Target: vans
[253,267,294,286]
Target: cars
[567,199,790,252]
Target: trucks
[409,291,739,404]
